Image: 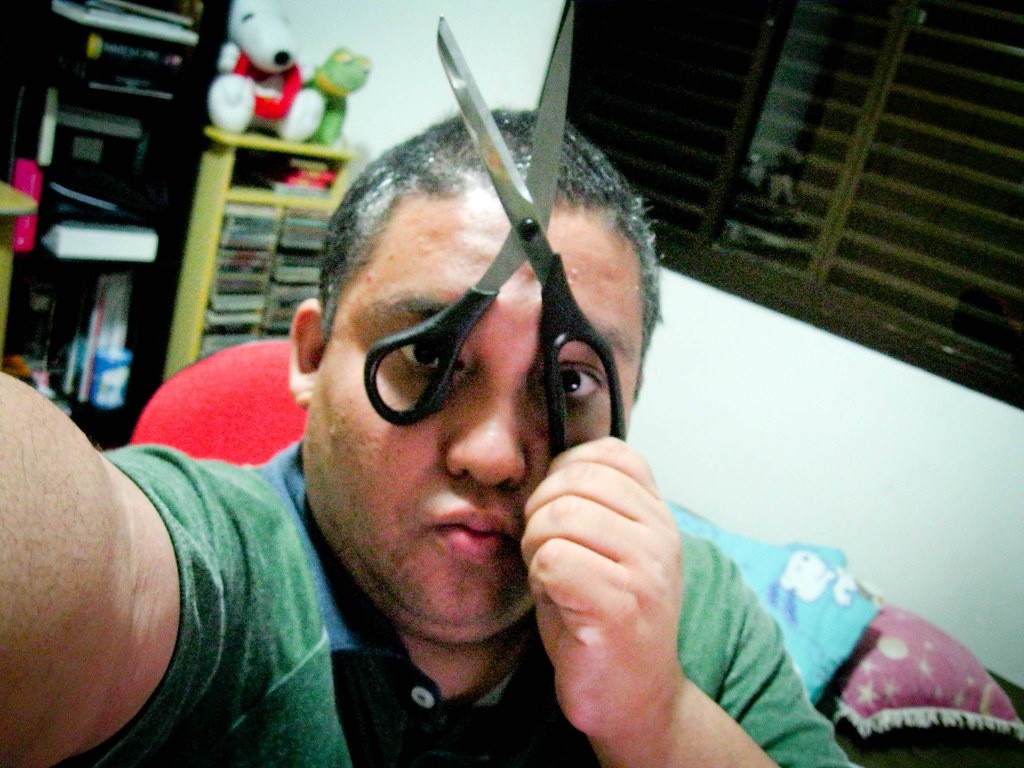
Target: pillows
[667,500,1024,743]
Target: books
[198,197,332,364]
[265,157,339,195]
[61,266,133,403]
[51,0,198,47]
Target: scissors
[363,0,629,458]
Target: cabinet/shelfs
[0,0,348,448]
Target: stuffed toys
[301,47,372,146]
[208,0,326,143]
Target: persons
[0,108,863,767]
